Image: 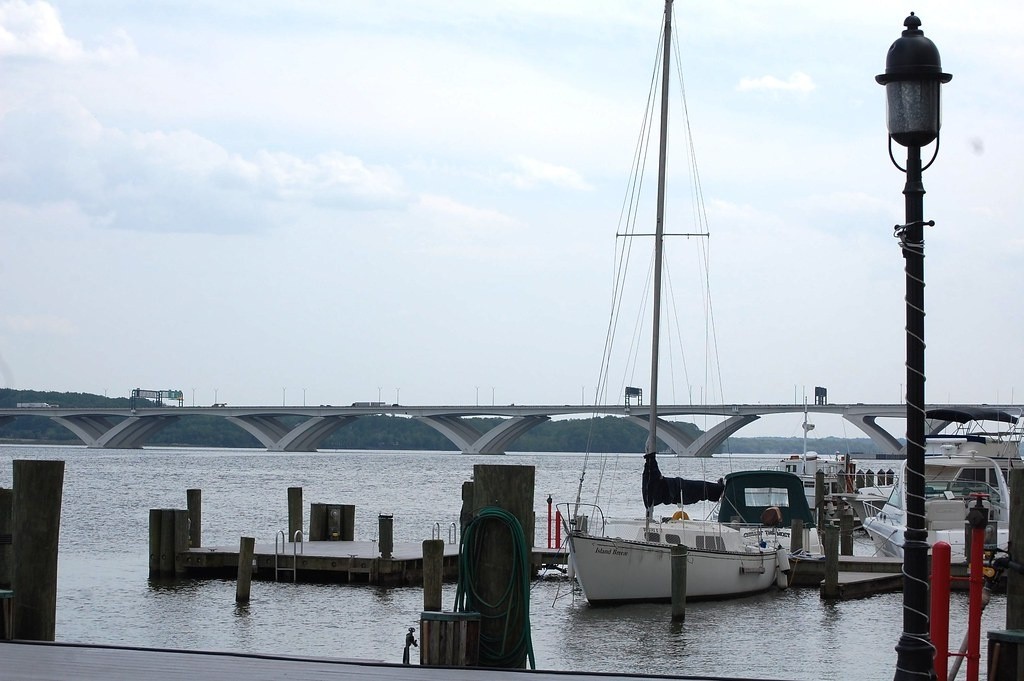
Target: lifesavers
[672,510,689,521]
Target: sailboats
[555,0,793,611]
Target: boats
[711,383,1024,551]
[861,437,1011,564]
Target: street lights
[302,387,308,406]
[377,387,383,407]
[793,384,798,405]
[282,386,287,406]
[873,10,953,681]
[491,387,496,406]
[899,384,903,405]
[213,388,219,404]
[396,388,401,406]
[699,385,704,406]
[192,388,196,408]
[476,386,480,406]
[688,385,693,406]
[802,384,806,405]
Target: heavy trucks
[16,402,51,408]
